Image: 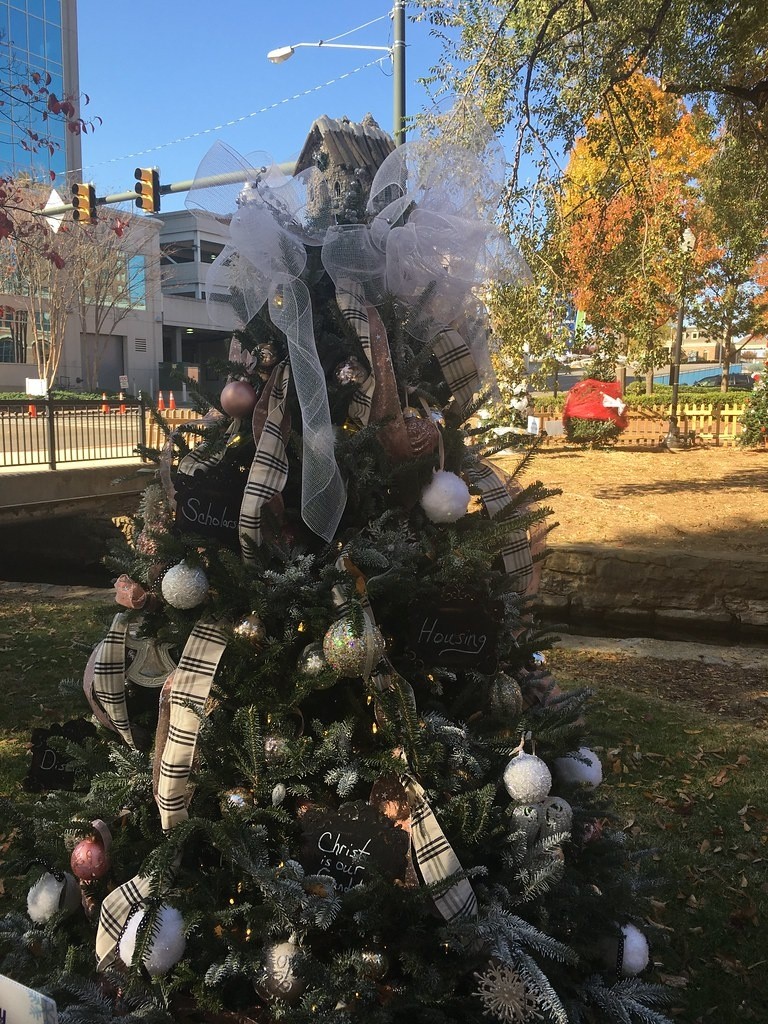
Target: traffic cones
[100,392,111,414]
[157,391,166,411]
[28,396,37,418]
[116,392,127,414]
[168,391,177,410]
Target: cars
[659,346,688,364]
[693,373,756,393]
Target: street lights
[268,42,404,190]
[660,222,697,448]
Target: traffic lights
[71,184,90,224]
[135,165,160,214]
